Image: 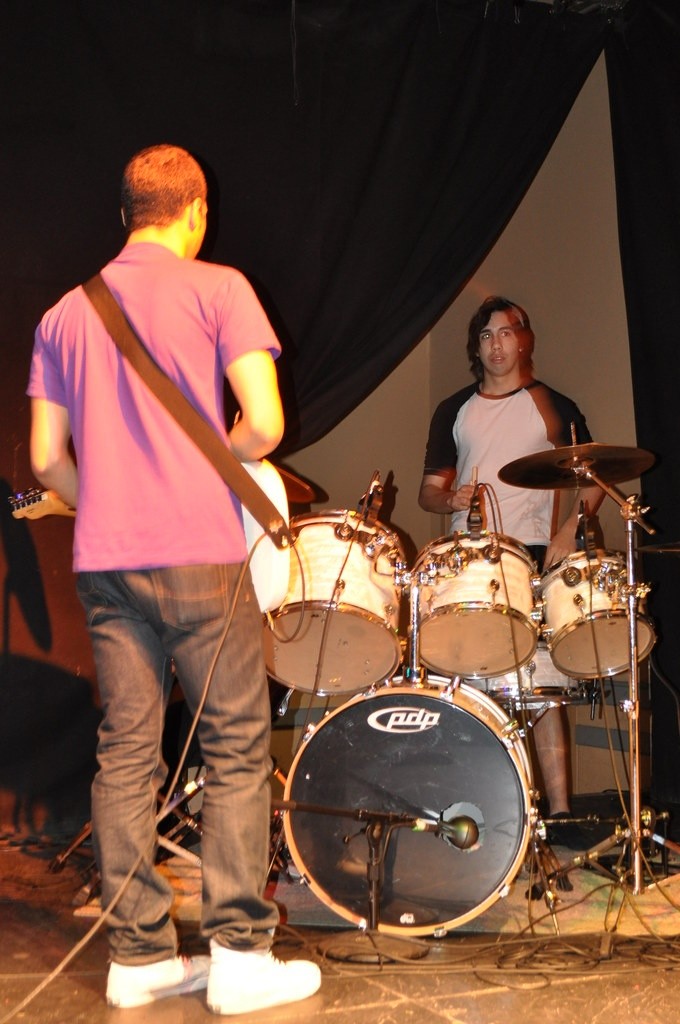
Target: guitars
[0,456,293,614]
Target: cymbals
[269,462,319,506]
[496,441,655,490]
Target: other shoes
[544,812,594,853]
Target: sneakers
[102,951,213,1007]
[203,937,322,1012]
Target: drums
[457,630,601,709]
[276,674,541,935]
[260,510,408,696]
[537,551,654,681]
[408,533,541,680]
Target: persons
[417,296,609,848]
[25,142,323,1017]
